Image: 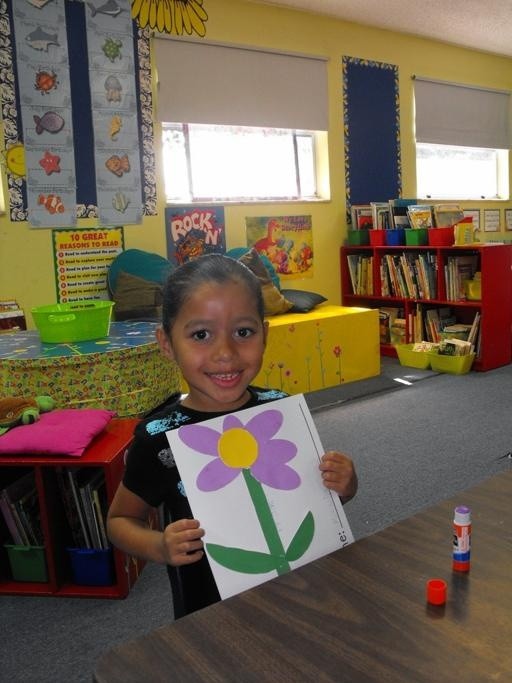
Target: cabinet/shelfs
[340,244,512,373]
[0,417,160,599]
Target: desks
[93,470,512,683]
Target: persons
[105,255,358,617]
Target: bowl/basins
[30,298,116,344]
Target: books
[59,468,109,565]
[379,306,480,368]
[347,254,478,301]
[1,479,40,550]
[351,199,465,230]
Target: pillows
[107,247,328,322]
[0,409,118,458]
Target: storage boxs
[369,228,385,246]
[347,229,366,245]
[404,227,427,246]
[428,228,454,246]
[385,227,403,245]
